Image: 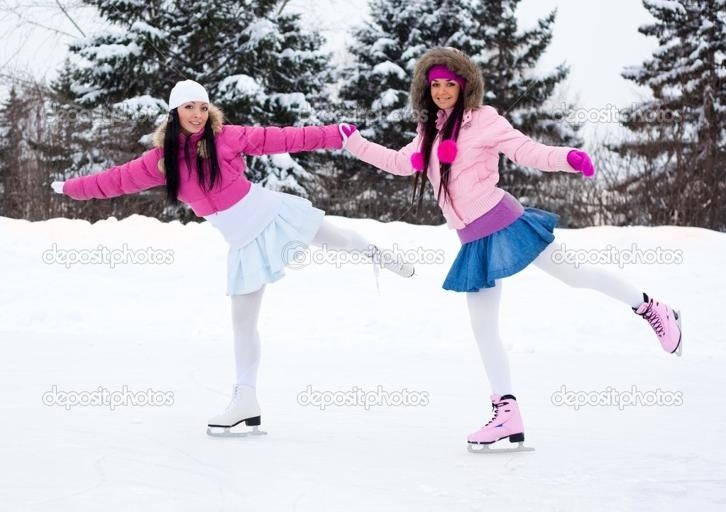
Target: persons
[340,46,682,445]
[51,78,416,429]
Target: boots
[207,385,260,427]
[366,246,413,278]
[632,293,681,353]
[467,394,526,444]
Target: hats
[169,80,209,112]
[428,66,465,85]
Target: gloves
[568,151,595,177]
[51,181,65,193]
[338,123,356,149]
